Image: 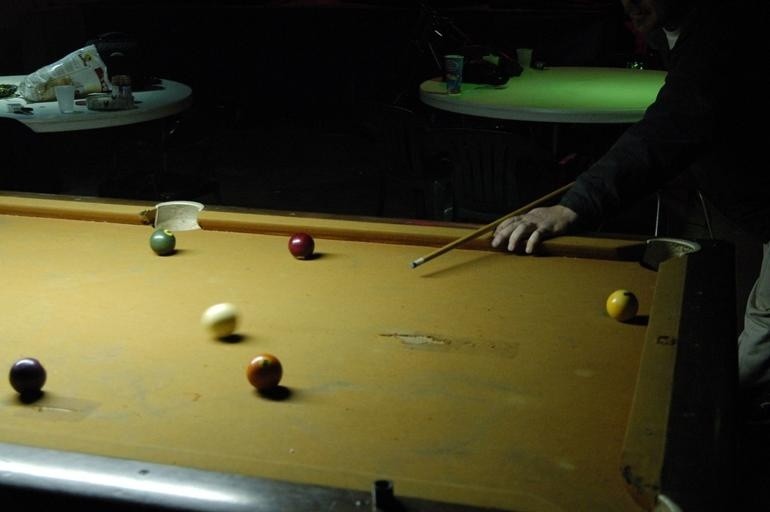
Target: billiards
[606,289,638,321]
[289,232,314,260]
[246,354,282,392]
[202,303,241,340]
[150,228,176,255]
[9,358,46,394]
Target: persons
[490,0,770,510]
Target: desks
[0,74,193,134]
[421,66,667,201]
[0,192,701,511]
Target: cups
[111,74,131,100]
[517,49,534,70]
[443,54,464,94]
[55,85,76,114]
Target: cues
[411,181,579,269]
